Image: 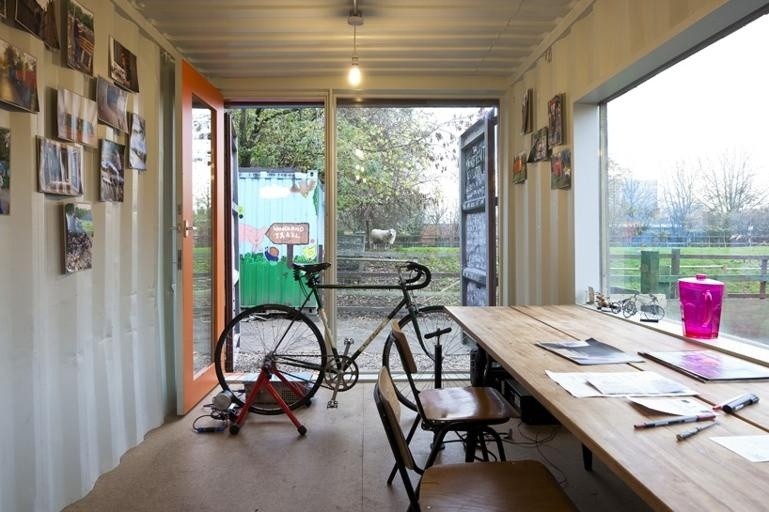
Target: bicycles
[214,261,484,415]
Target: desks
[443,304,768,512]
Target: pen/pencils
[633,413,717,431]
[712,391,745,410]
[676,422,715,441]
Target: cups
[675,272,726,340]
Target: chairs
[373,320,581,511]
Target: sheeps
[369,227,397,250]
